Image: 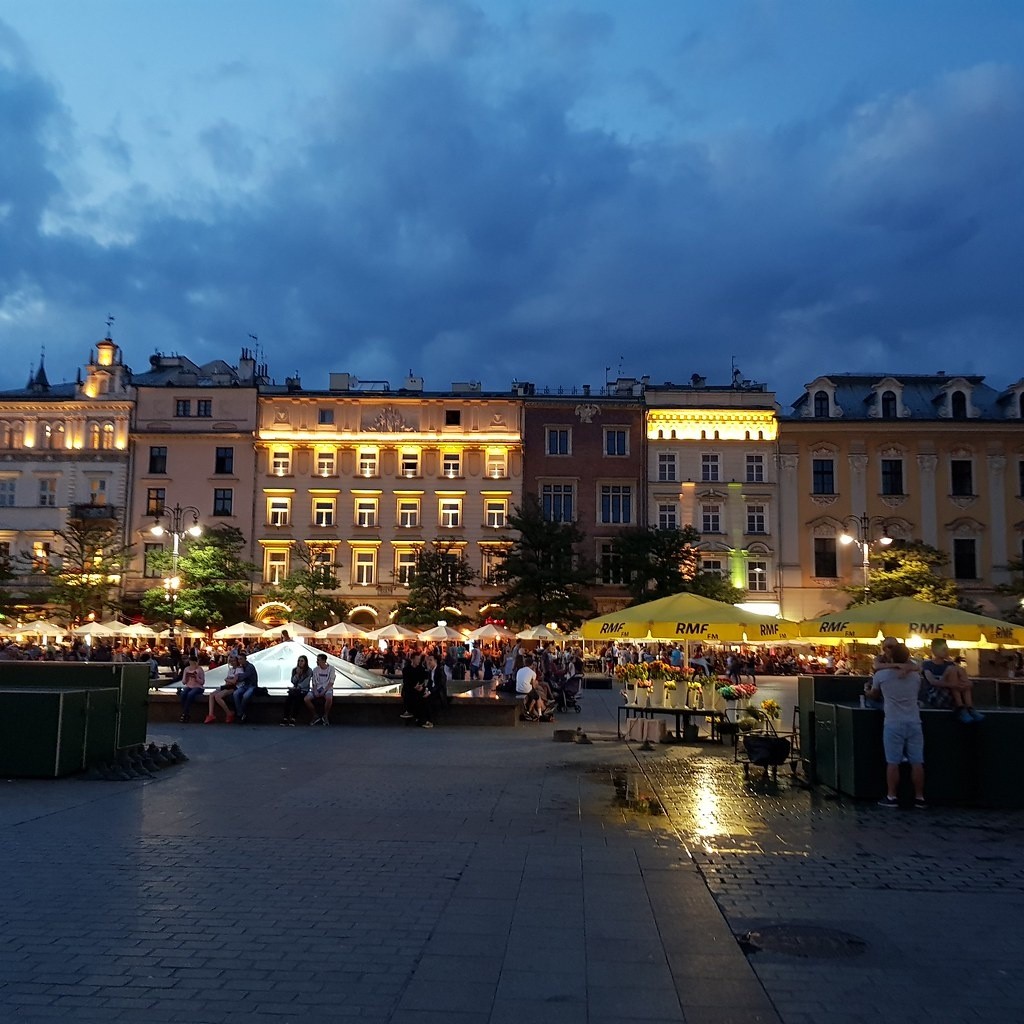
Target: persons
[3,629,580,726]
[689,641,835,687]
[602,639,685,672]
[862,636,926,808]
[920,636,985,723]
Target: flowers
[615,660,759,701]
[714,722,739,735]
[760,698,783,720]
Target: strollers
[554,672,584,713]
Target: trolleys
[725,708,801,774]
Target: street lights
[151,502,202,652]
[840,512,894,605]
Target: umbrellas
[10,620,68,645]
[69,622,112,647]
[578,592,798,679]
[113,621,157,648]
[262,622,316,640]
[468,624,516,648]
[516,624,568,649]
[367,623,418,646]
[316,622,367,647]
[0,623,12,636]
[213,621,263,643]
[159,624,206,649]
[793,594,1024,650]
[418,626,468,654]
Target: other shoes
[280,718,288,726]
[953,704,975,724]
[142,751,160,771]
[237,714,248,724]
[158,744,176,763]
[422,721,434,728]
[288,720,297,726]
[100,749,153,780]
[170,742,191,760]
[524,699,556,722]
[400,711,413,718]
[967,706,986,721]
[179,713,185,722]
[147,743,168,768]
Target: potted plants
[740,705,764,733]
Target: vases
[624,681,749,722]
[722,733,732,746]
[768,719,781,731]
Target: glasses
[886,644,896,649]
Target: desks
[618,706,724,743]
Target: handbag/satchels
[253,687,269,697]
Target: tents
[566,630,615,656]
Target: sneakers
[204,714,216,724]
[322,716,329,726]
[224,711,235,724]
[877,795,899,807]
[310,716,320,727]
[915,798,929,808]
